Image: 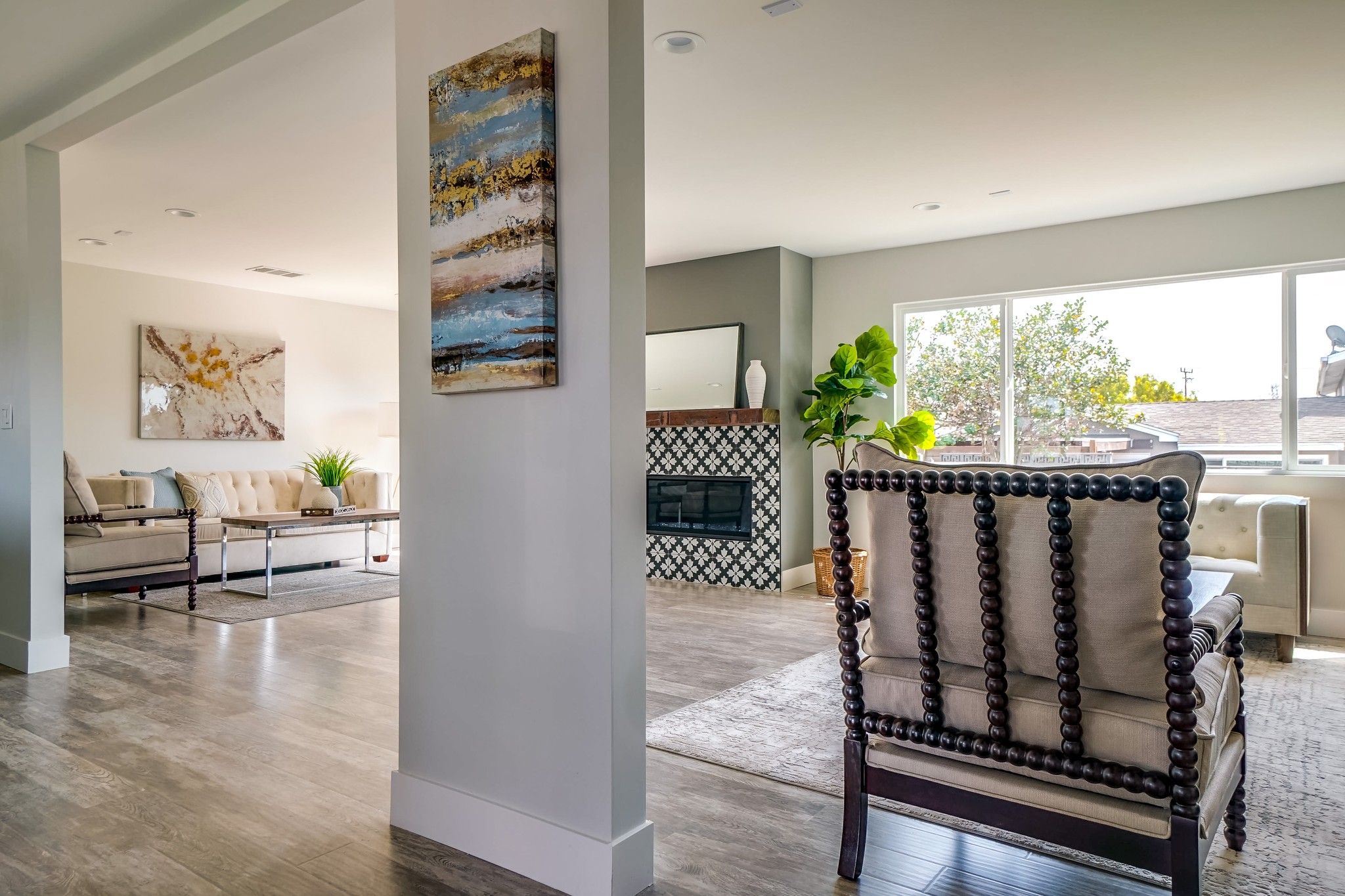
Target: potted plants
[290,446,377,505]
[800,326,936,596]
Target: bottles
[745,360,766,408]
[311,487,339,509]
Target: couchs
[1183,491,1311,662]
[87,469,391,609]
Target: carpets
[647,642,1345,896]
[109,564,399,624]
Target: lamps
[378,402,400,439]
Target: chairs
[826,439,1248,896]
[64,450,199,611]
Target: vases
[745,360,766,409]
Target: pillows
[175,471,230,518]
[119,466,185,514]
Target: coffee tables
[1188,569,1234,619]
[221,508,400,599]
